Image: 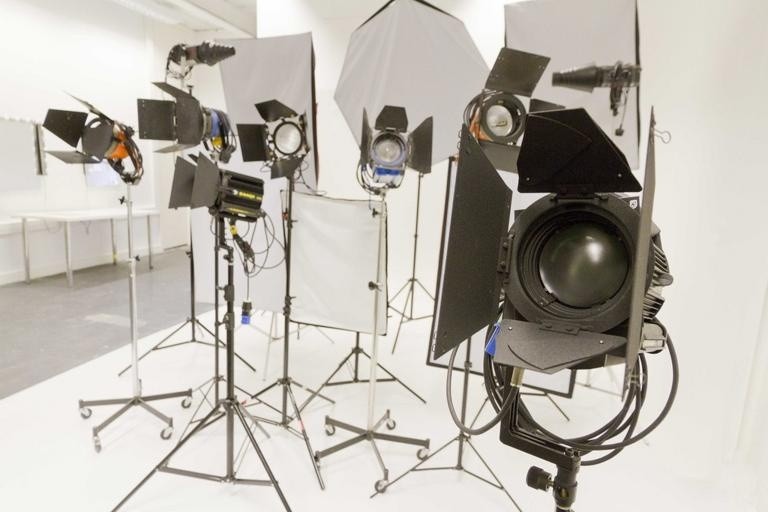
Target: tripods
[111,171,522,512]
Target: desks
[11,207,162,284]
